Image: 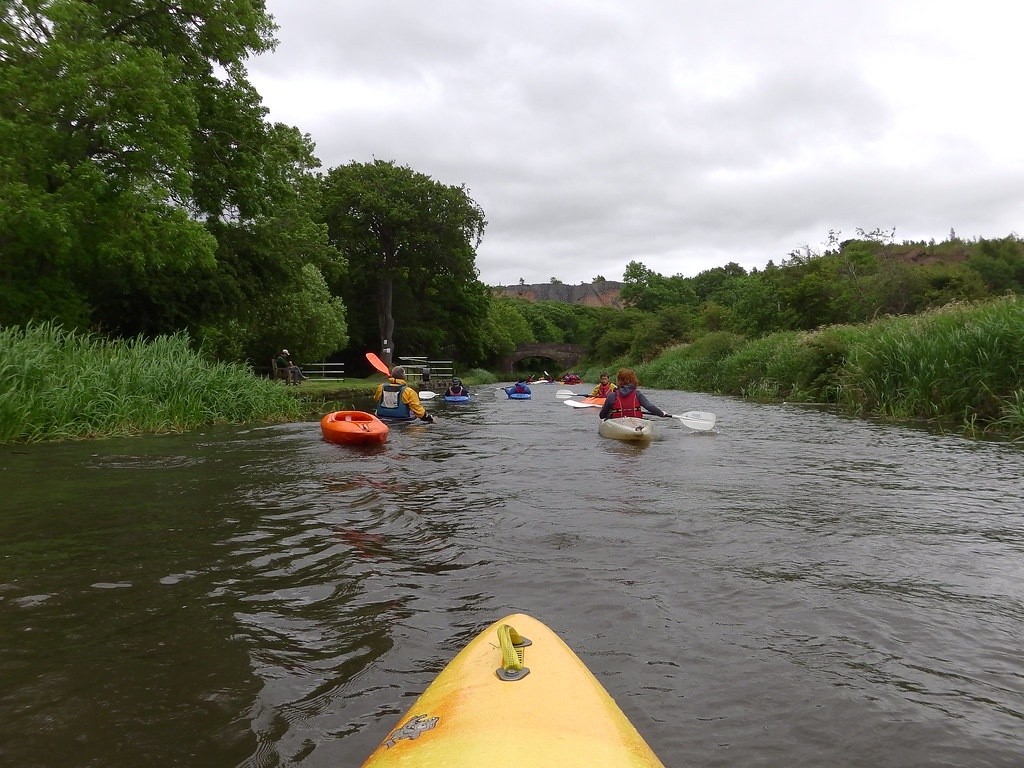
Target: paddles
[642,410,718,431]
[555,389,596,399]
[530,374,536,380]
[544,370,550,377]
[494,386,514,392]
[419,390,445,400]
[366,352,393,377]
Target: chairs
[272,359,301,385]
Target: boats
[374,410,418,426]
[579,396,608,405]
[436,396,474,408]
[508,393,533,401]
[321,410,390,445]
[599,417,657,443]
[528,379,582,387]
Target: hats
[283,349,290,355]
[453,379,459,384]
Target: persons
[444,378,470,396]
[600,368,667,423]
[591,372,618,398]
[527,371,581,383]
[373,366,433,423]
[502,377,531,396]
[276,349,309,386]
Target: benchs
[301,363,346,382]
[400,361,454,381]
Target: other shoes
[302,376,309,381]
